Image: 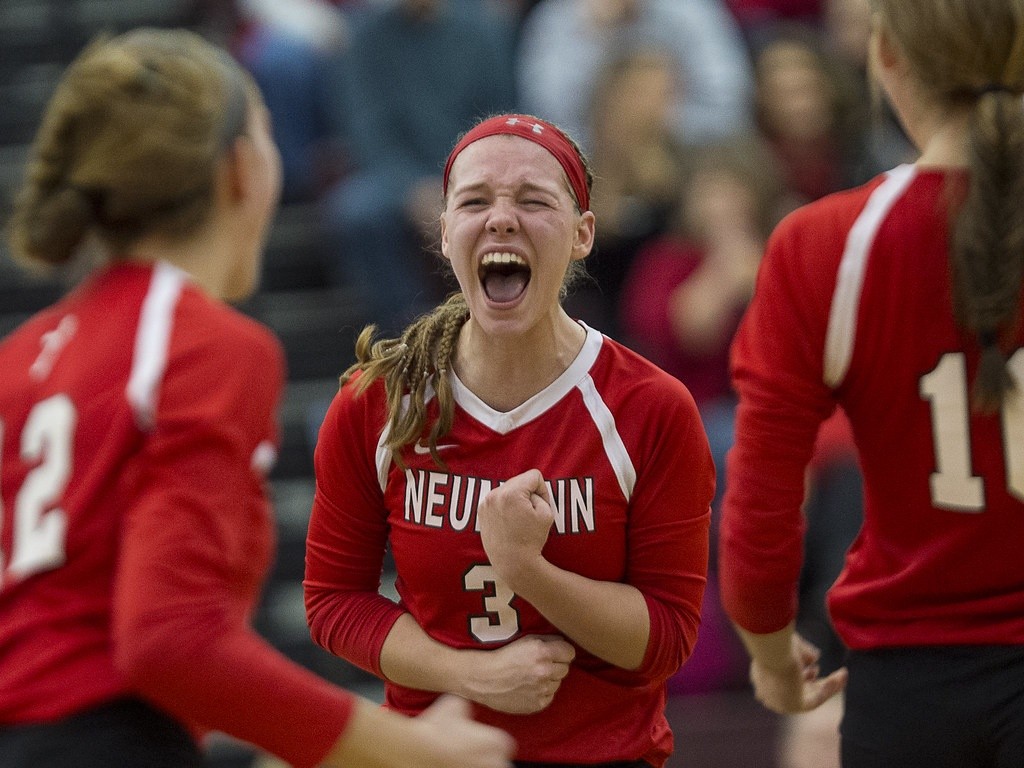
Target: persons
[0,26,513,768]
[302,116,717,768]
[0,0,1024,768]
[720,0,1024,768]
